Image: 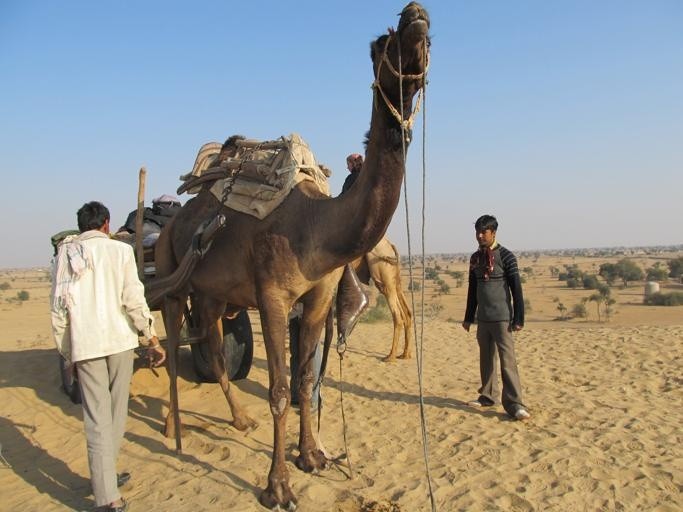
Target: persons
[51,201,168,512]
[460,215,532,419]
[338,152,365,198]
[286,302,322,412]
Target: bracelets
[149,344,160,350]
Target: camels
[351,235,413,362]
[154,0,431,512]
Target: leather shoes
[114,472,130,486]
[108,495,127,512]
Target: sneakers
[467,396,481,407]
[512,409,531,419]
